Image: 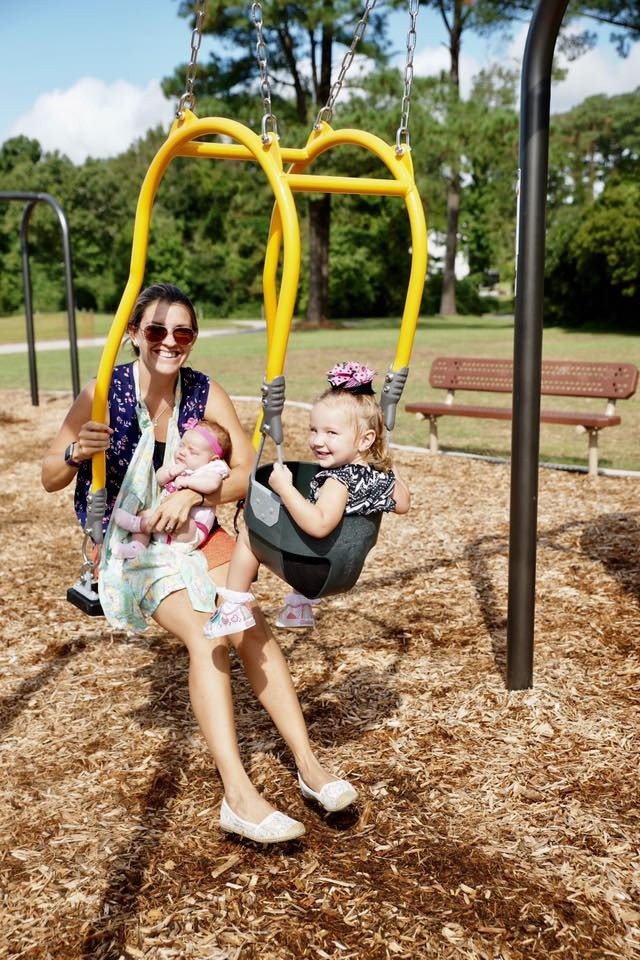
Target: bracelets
[198,493,204,506]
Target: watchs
[65,442,80,468]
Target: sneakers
[275,604,314,628]
[204,606,256,638]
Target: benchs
[405,356,640,483]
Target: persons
[202,362,411,639]
[41,284,358,843]
[112,420,231,559]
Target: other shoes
[298,768,359,811]
[219,796,306,843]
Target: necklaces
[146,405,170,426]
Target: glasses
[138,326,196,345]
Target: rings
[166,525,174,530]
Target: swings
[65,0,427,620]
